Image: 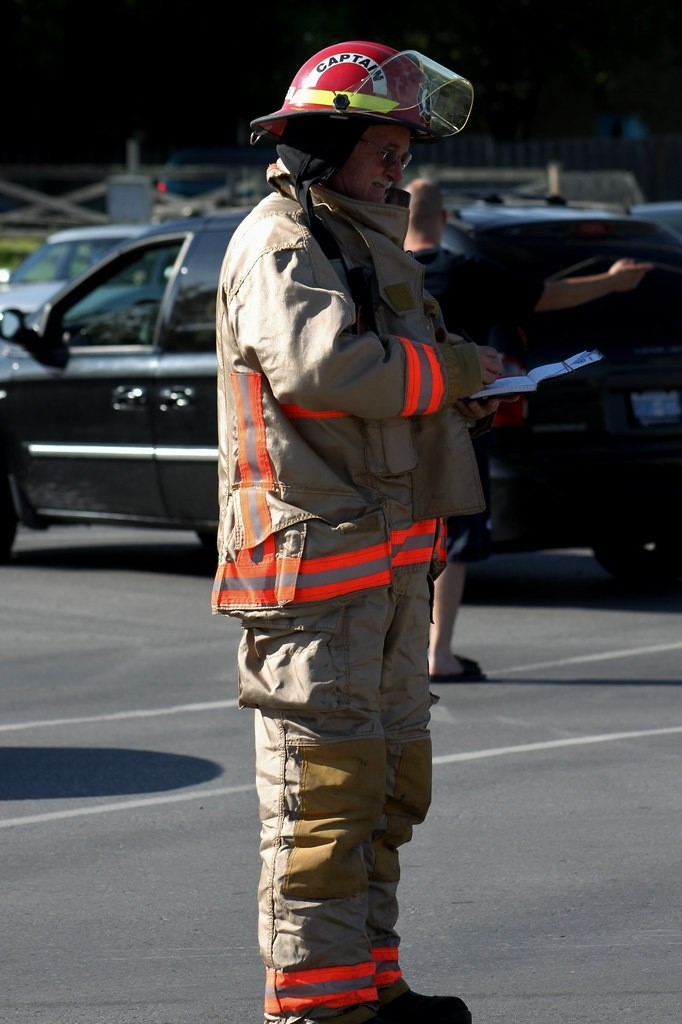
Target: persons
[399,178,655,683]
[211,42,522,1024]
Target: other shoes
[364,990,472,1024]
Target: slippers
[428,653,489,685]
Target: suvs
[422,191,681,590]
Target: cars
[0,209,258,575]
[1,159,682,274]
[0,224,183,366]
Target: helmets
[250,41,443,146]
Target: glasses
[359,137,413,173]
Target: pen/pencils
[461,330,502,376]
